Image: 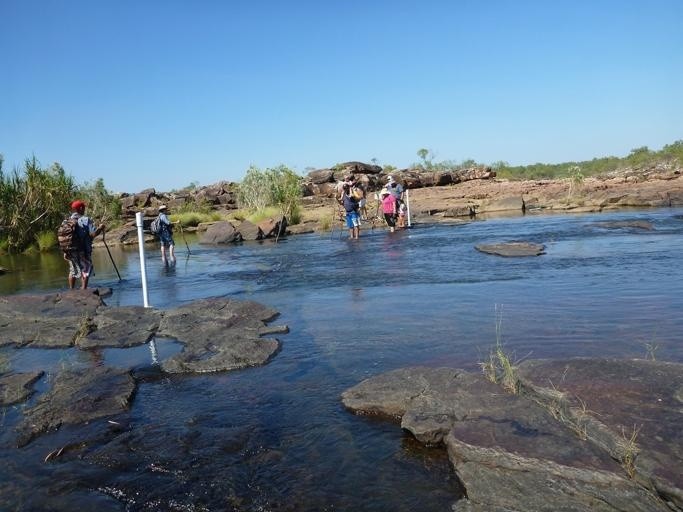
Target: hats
[158,205,168,211]
[72,201,85,209]
[380,188,390,194]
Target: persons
[354,183,370,221]
[63,200,105,290]
[343,230,409,265]
[384,180,407,228]
[336,183,361,241]
[160,264,179,304]
[342,176,356,188]
[155,204,181,266]
[378,187,397,233]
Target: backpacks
[58,215,82,252]
[151,214,163,235]
